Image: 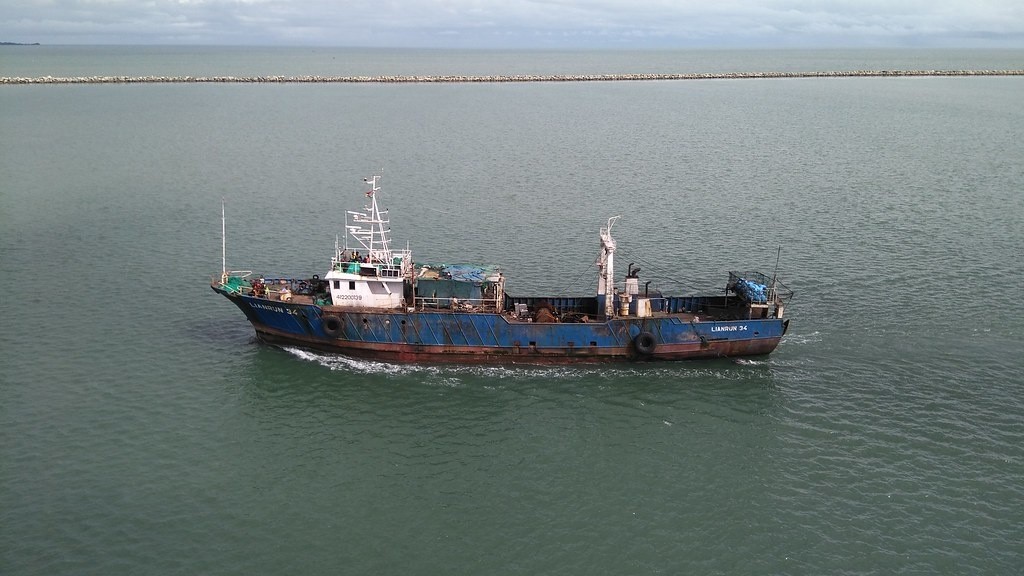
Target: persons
[367,254,370,263]
[342,248,360,262]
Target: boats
[209,172,796,361]
